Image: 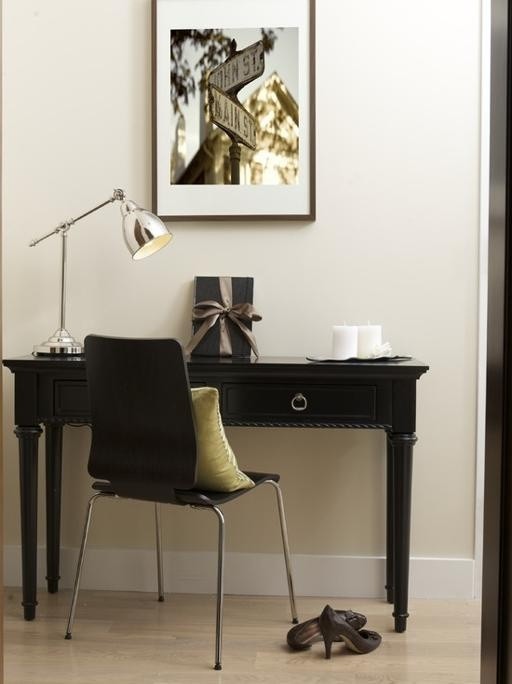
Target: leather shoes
[285,609,367,651]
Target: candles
[332,325,357,360]
[358,325,382,358]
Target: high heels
[317,603,382,659]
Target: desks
[0,357,430,633]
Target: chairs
[64,334,299,670]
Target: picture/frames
[150,0,316,222]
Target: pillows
[191,387,256,494]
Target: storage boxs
[191,276,254,357]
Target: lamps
[29,187,173,357]
